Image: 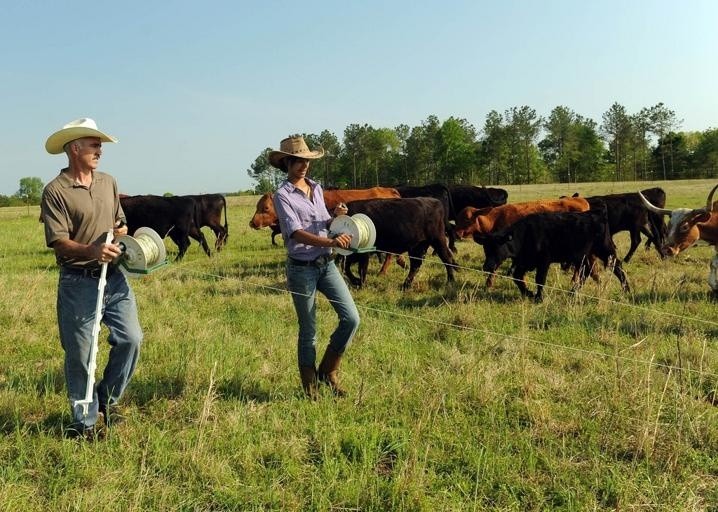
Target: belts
[287,251,337,268]
[60,261,118,279]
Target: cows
[473,205,632,297]
[559,188,669,264]
[186,193,229,252]
[249,183,508,288]
[637,182,717,260]
[119,194,212,262]
[455,195,591,294]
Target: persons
[266,133,361,401]
[38,114,145,446]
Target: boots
[316,344,348,396]
[299,364,323,402]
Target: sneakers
[72,424,107,442]
[98,403,127,427]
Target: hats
[269,134,325,171]
[46,116,118,155]
